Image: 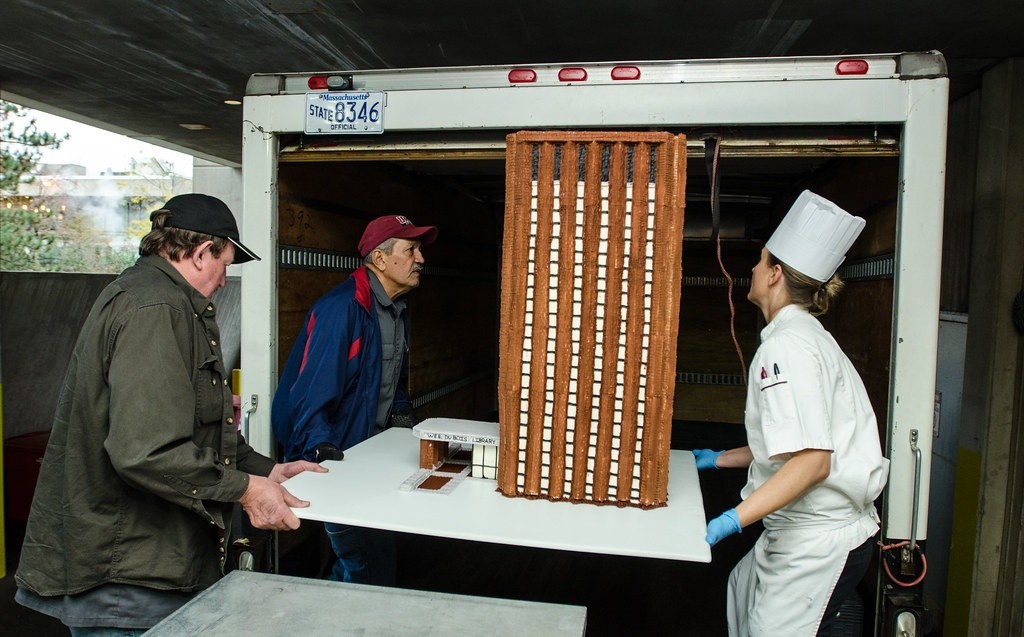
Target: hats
[766,189,867,281]
[359,215,438,258]
[162,193,261,265]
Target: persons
[13,193,329,637]
[271,214,436,585]
[691,189,889,637]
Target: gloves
[314,443,344,464]
[692,448,725,469]
[706,508,742,548]
